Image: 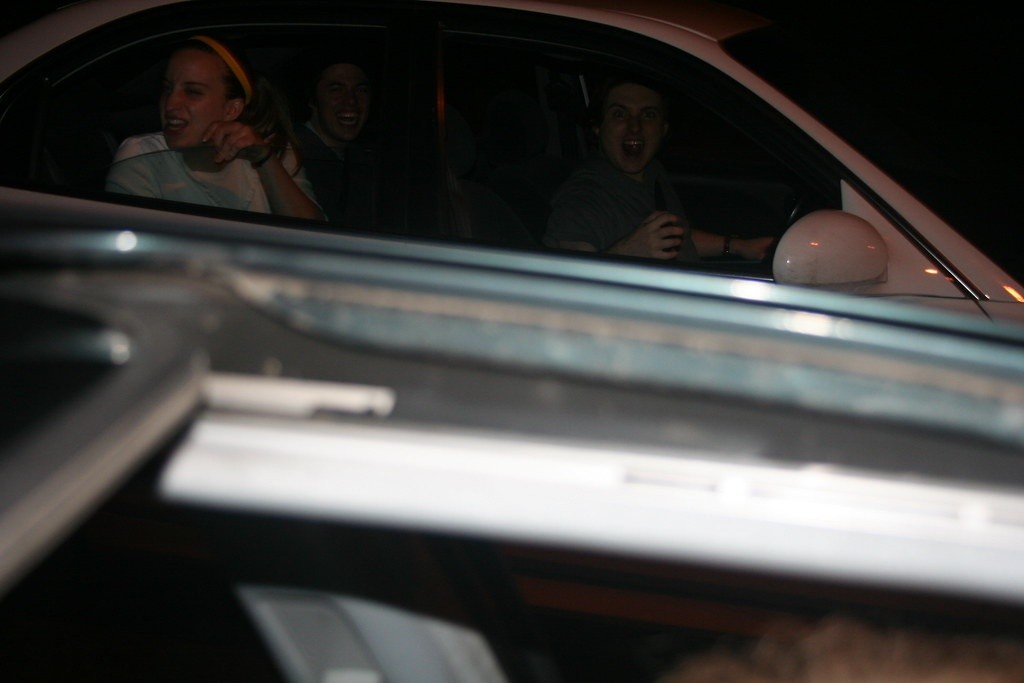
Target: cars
[1,1,1024,448]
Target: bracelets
[251,145,273,169]
[724,236,730,254]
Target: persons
[541,72,781,258]
[289,57,409,237]
[105,36,323,220]
